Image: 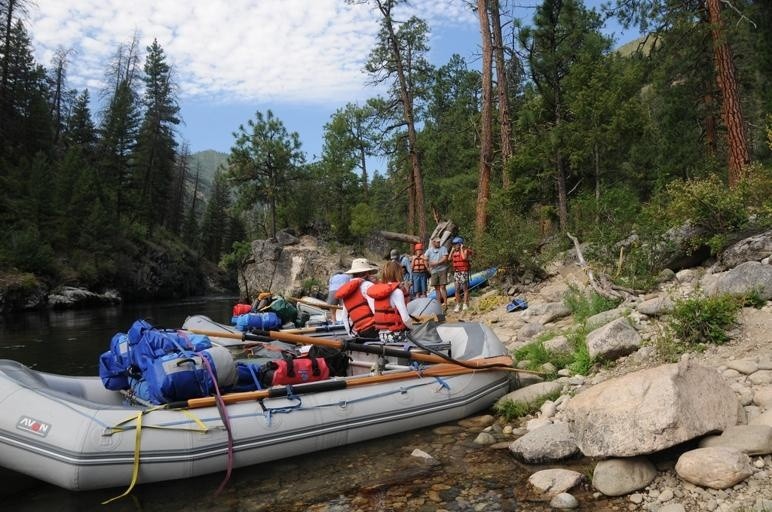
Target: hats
[452,236,464,244]
[344,257,379,274]
[415,243,424,251]
[390,249,400,259]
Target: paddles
[187,327,543,408]
[286,296,439,326]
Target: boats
[0,297,518,493]
[428,252,517,300]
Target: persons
[327,236,473,344]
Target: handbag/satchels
[259,294,297,325]
[261,349,328,390]
[306,345,350,377]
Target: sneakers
[453,303,469,313]
